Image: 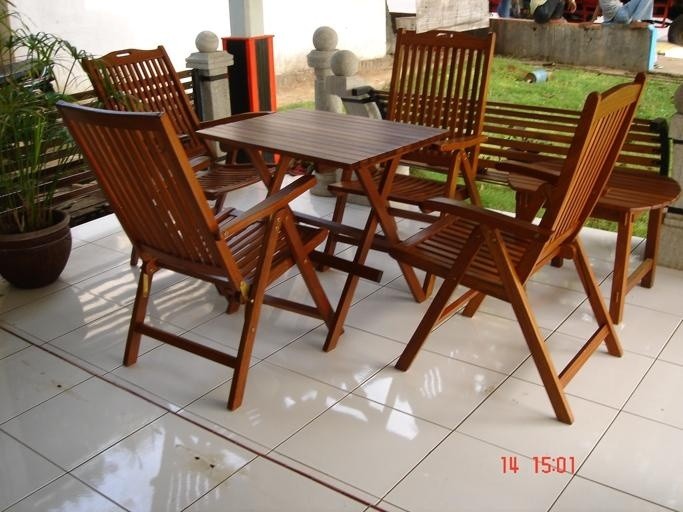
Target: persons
[529,0,577,23]
[591,0,653,29]
[497,0,522,17]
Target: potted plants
[0,0,150,289]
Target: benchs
[352,86,671,187]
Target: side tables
[508,163,682,323]
